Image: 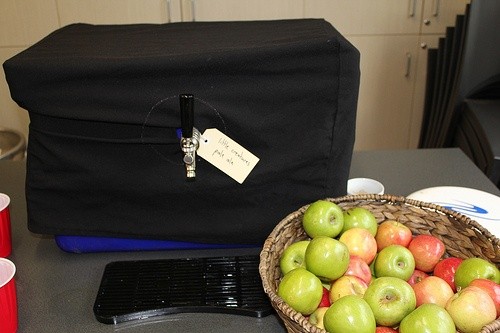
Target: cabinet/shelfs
[0,0,477,151]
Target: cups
[347,178,384,203]
[0,193,13,256]
[0,256,19,333]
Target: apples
[276,201,500,333]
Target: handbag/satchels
[3,18,362,244]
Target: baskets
[259,194,500,333]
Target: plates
[405,185,500,243]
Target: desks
[0,150,500,333]
